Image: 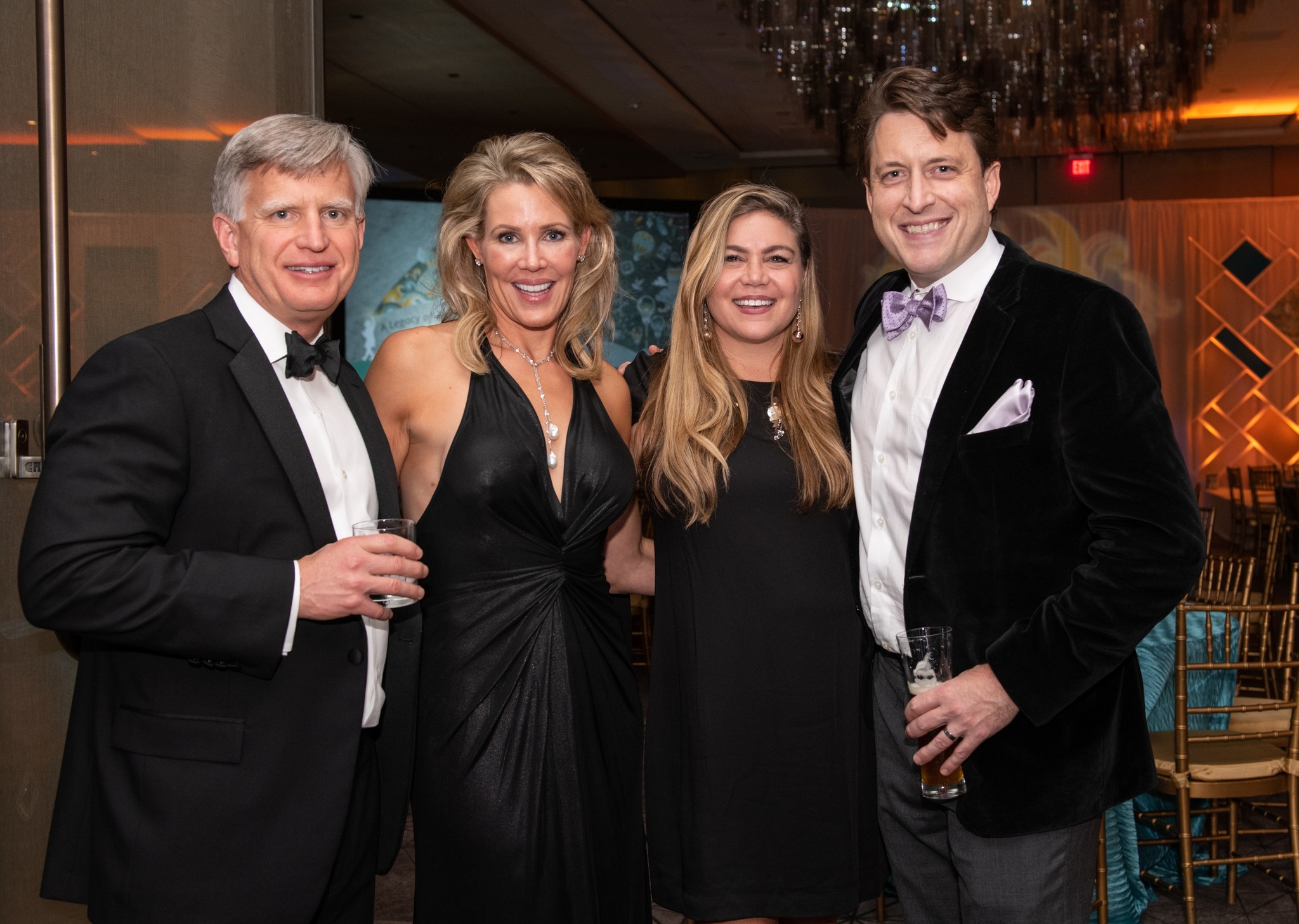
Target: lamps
[736,0,1247,179]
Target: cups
[895,626,968,799]
[351,518,418,608]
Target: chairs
[1091,461,1299,924]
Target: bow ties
[880,284,948,341]
[285,330,342,385]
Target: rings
[943,726,959,743]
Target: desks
[1090,601,1242,924]
[1202,485,1295,553]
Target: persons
[615,183,894,924]
[360,131,659,924]
[15,113,429,924]
[832,62,1210,924]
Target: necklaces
[492,328,560,470]
